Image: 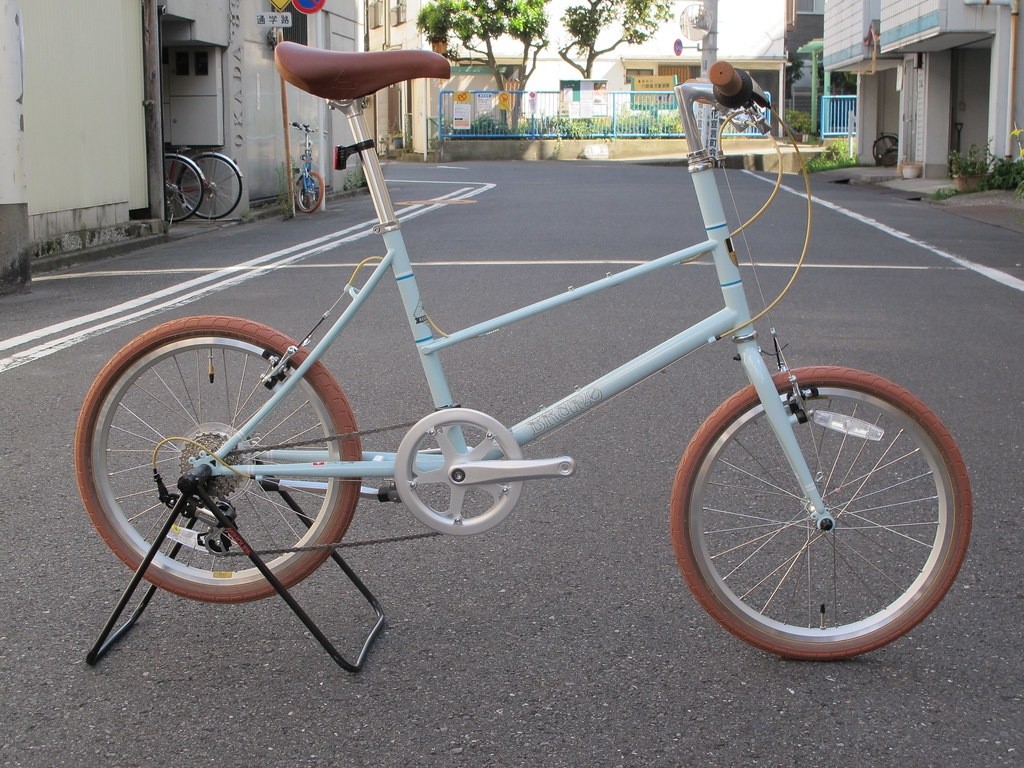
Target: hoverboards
[1010,121,1024,163]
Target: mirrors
[679,3,713,41]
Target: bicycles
[164,143,244,220]
[161,143,206,227]
[289,122,328,213]
[74,40,973,660]
[872,132,899,167]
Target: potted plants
[947,134,993,194]
